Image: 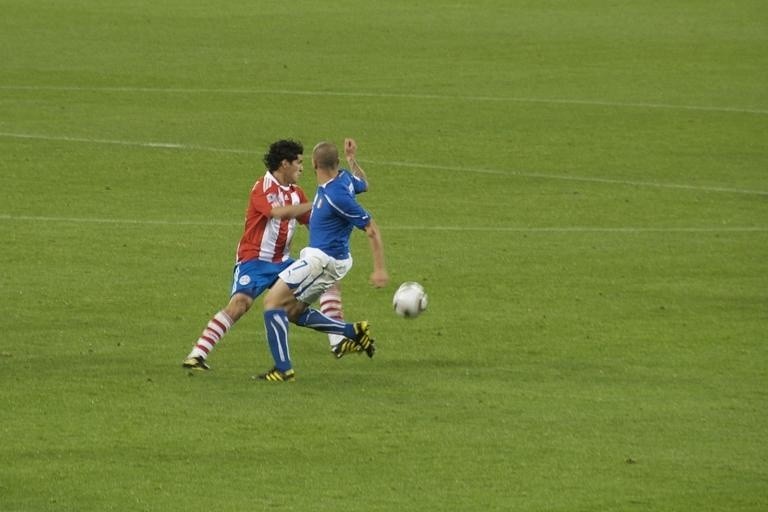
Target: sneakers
[182,357,211,372]
[330,339,363,358]
[354,321,375,358]
[251,369,294,382]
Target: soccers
[393,282,428,318]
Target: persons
[248,137,390,384]
[179,136,365,374]
